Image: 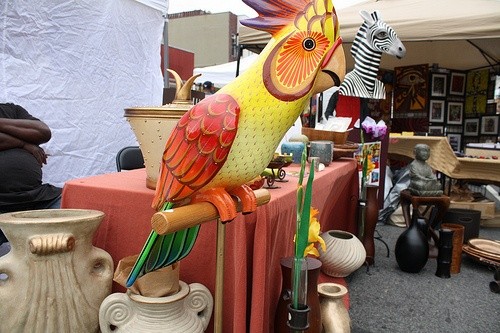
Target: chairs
[116,145,144,171]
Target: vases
[290,258,308,312]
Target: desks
[400,190,451,232]
[60,149,358,333]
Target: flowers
[292,141,327,261]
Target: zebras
[339,9,407,100]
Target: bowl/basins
[309,140,334,167]
[280,141,306,166]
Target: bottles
[395,209,429,274]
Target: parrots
[127,0,346,290]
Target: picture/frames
[428,69,500,154]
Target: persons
[0,104,64,243]
[410,144,440,191]
